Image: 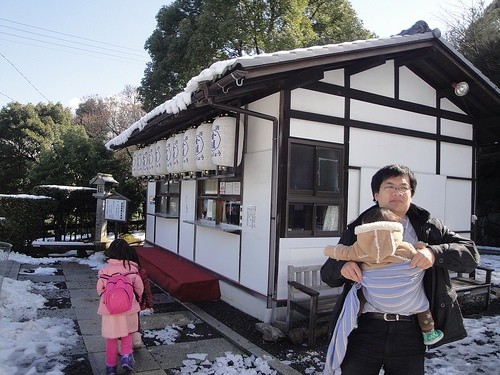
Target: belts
[367,313,411,322]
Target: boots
[117,340,121,355]
[132,331,142,352]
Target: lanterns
[131,130,185,180]
[184,125,200,177]
[211,109,244,172]
[195,119,217,175]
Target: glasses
[384,185,413,193]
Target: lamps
[452,82,470,97]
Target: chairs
[442,264,495,313]
[289,265,353,349]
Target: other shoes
[106,365,117,375]
[121,354,136,370]
[422,330,444,345]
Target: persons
[322,206,445,346]
[96,239,145,375]
[320,164,481,375]
[116,246,154,362]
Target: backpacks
[100,271,138,314]
